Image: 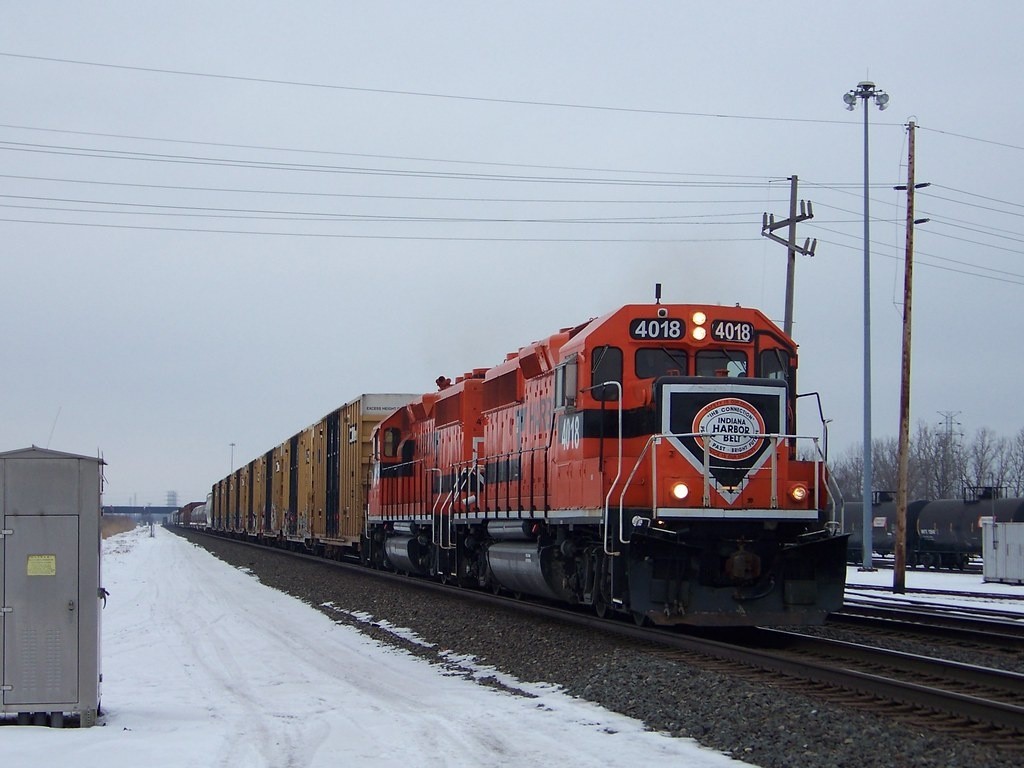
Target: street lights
[228,443,237,472]
[844,82,892,566]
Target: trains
[840,488,1024,569]
[162,283,852,621]
